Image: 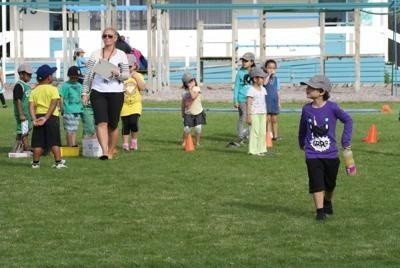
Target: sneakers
[324,196,333,216]
[31,160,41,169]
[51,160,71,170]
[122,144,129,151]
[99,155,109,160]
[130,140,137,150]
[316,208,326,220]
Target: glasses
[102,33,116,39]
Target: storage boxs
[80,137,104,157]
[49,145,81,158]
[8,150,32,158]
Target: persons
[296,75,354,221]
[230,51,256,147]
[73,48,92,75]
[12,62,35,151]
[81,28,131,160]
[59,67,90,147]
[0,60,8,109]
[243,66,270,156]
[262,60,283,142]
[179,72,207,149]
[27,64,67,169]
[80,78,98,139]
[120,52,146,151]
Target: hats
[126,53,137,66]
[249,66,267,78]
[240,52,255,61]
[67,65,83,78]
[300,74,332,93]
[17,63,33,74]
[182,72,195,83]
[36,64,57,80]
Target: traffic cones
[184,132,195,152]
[382,103,394,113]
[361,122,380,143]
[265,128,274,148]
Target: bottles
[239,127,248,138]
[342,146,357,175]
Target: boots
[181,132,201,147]
